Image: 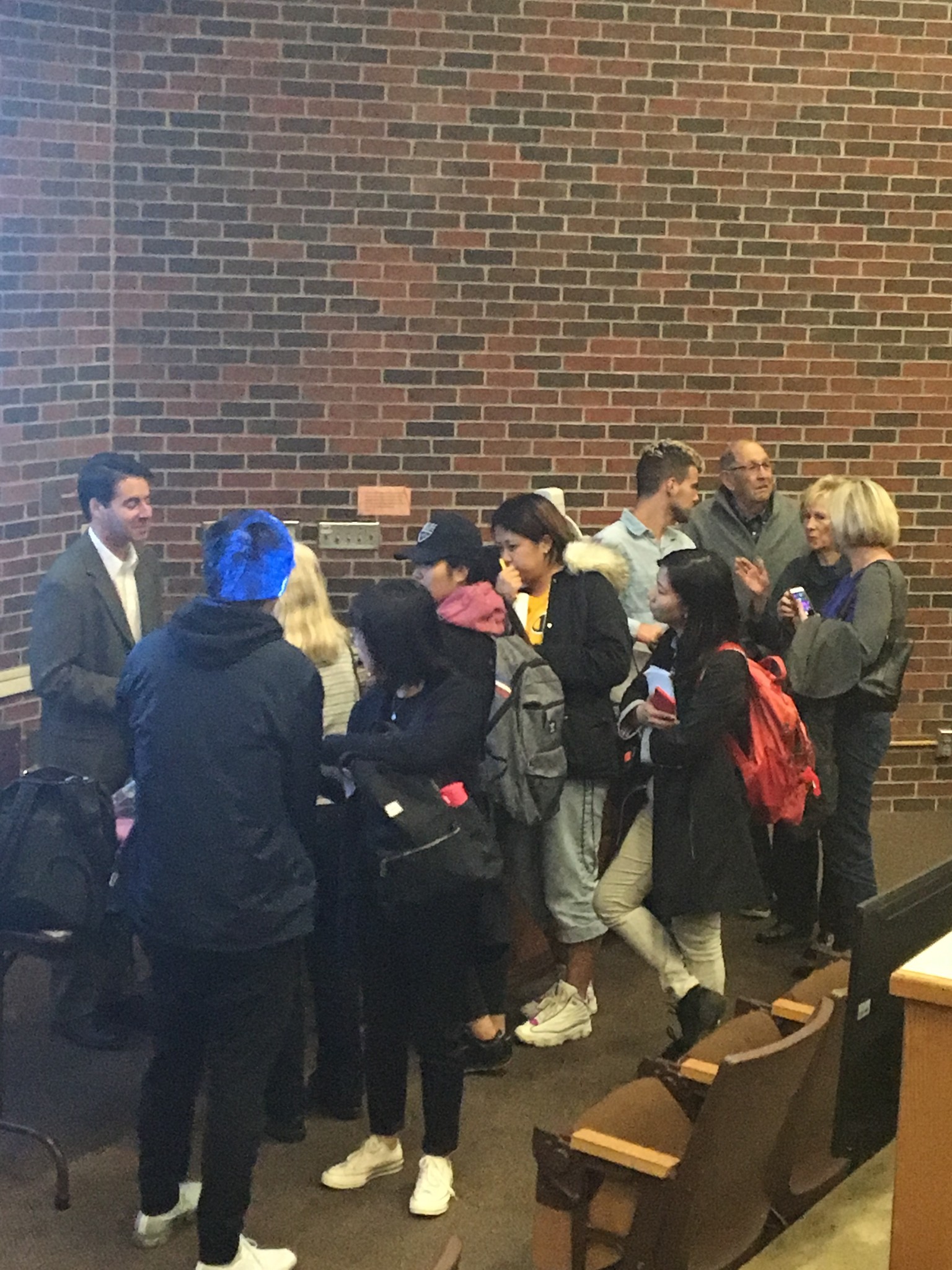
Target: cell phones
[788,586,816,617]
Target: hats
[395,512,483,564]
[534,487,582,539]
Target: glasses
[730,461,771,472]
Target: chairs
[528,945,853,1270]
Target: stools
[0,926,74,1213]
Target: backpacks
[476,629,566,825]
[717,641,820,825]
[0,768,116,929]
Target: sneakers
[456,1029,512,1074]
[793,932,844,980]
[663,985,728,1060]
[515,981,593,1047]
[65,1011,133,1047]
[305,1080,362,1121]
[319,1133,403,1188]
[408,1155,454,1216]
[496,1014,516,1048]
[134,1180,202,1245]
[195,1233,296,1270]
[740,895,777,918]
[754,916,812,945]
[264,1112,309,1143]
[523,981,599,1017]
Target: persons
[264,435,907,1215]
[29,453,166,1049]
[112,509,324,1270]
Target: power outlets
[283,521,303,543]
[319,521,382,551]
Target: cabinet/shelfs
[887,927,952,1270]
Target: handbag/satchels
[342,759,503,901]
